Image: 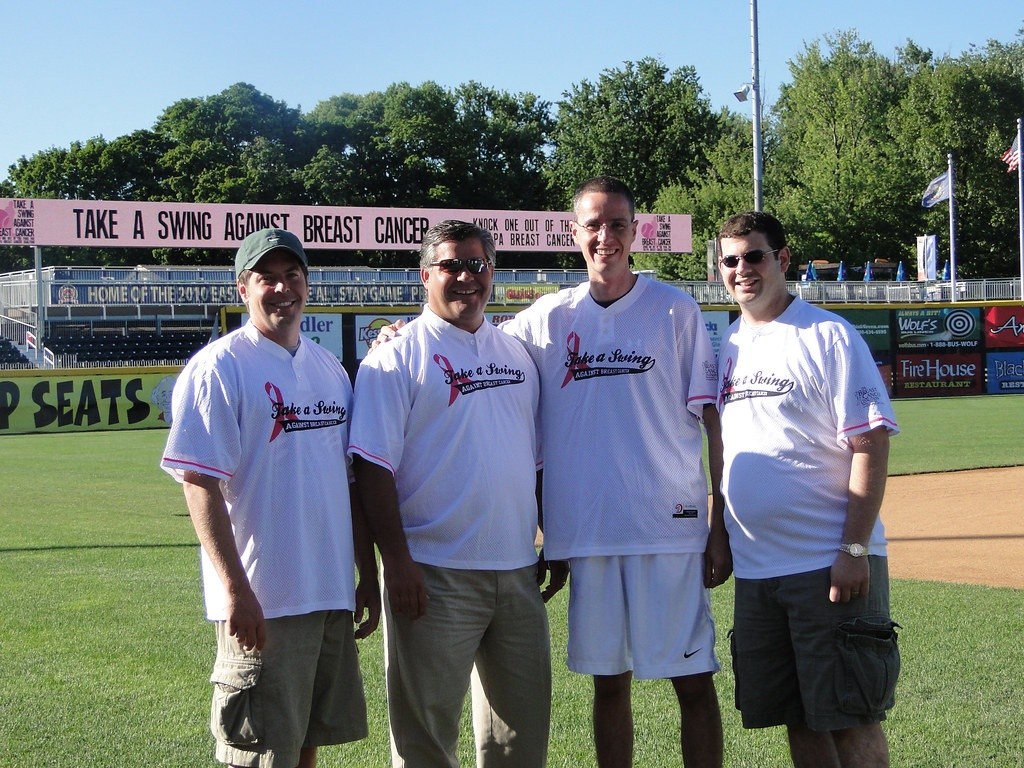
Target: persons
[348,219,569,768]
[370,177,733,768]
[716,211,899,768]
[161,229,381,768]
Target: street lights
[733,81,765,215]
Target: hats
[235,229,308,279]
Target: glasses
[429,258,491,276]
[574,220,633,233]
[720,249,779,268]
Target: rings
[561,581,565,585]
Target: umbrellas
[895,263,906,281]
[943,262,950,280]
[863,263,874,281]
[806,263,815,281]
[837,262,848,281]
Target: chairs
[0,327,219,370]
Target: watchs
[840,543,868,557]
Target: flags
[923,172,950,207]
[1003,134,1019,170]
